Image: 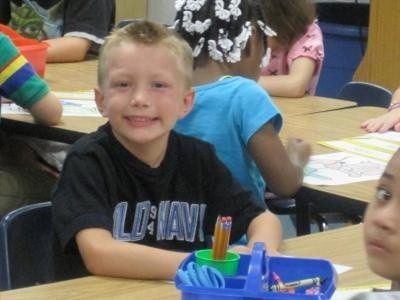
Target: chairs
[265,80,394,232]
[2,202,53,290]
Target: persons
[0,0,115,64]
[174,1,311,246]
[363,87,400,134]
[0,32,63,221]
[341,148,400,300]
[37,19,281,281]
[243,0,324,97]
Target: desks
[1,222,392,299]
[276,105,399,237]
[1,54,357,146]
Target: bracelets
[388,102,400,111]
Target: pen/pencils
[213,215,232,260]
[270,271,326,292]
